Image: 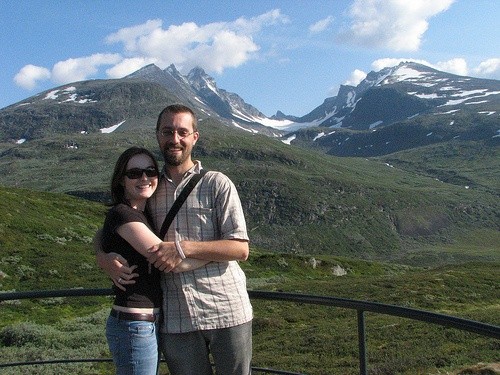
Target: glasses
[156,129,194,137]
[124,166,157,180]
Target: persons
[94,104,253,375]
[102,147,212,375]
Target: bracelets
[175,241,187,260]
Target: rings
[118,278,121,283]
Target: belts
[109,308,156,322]
